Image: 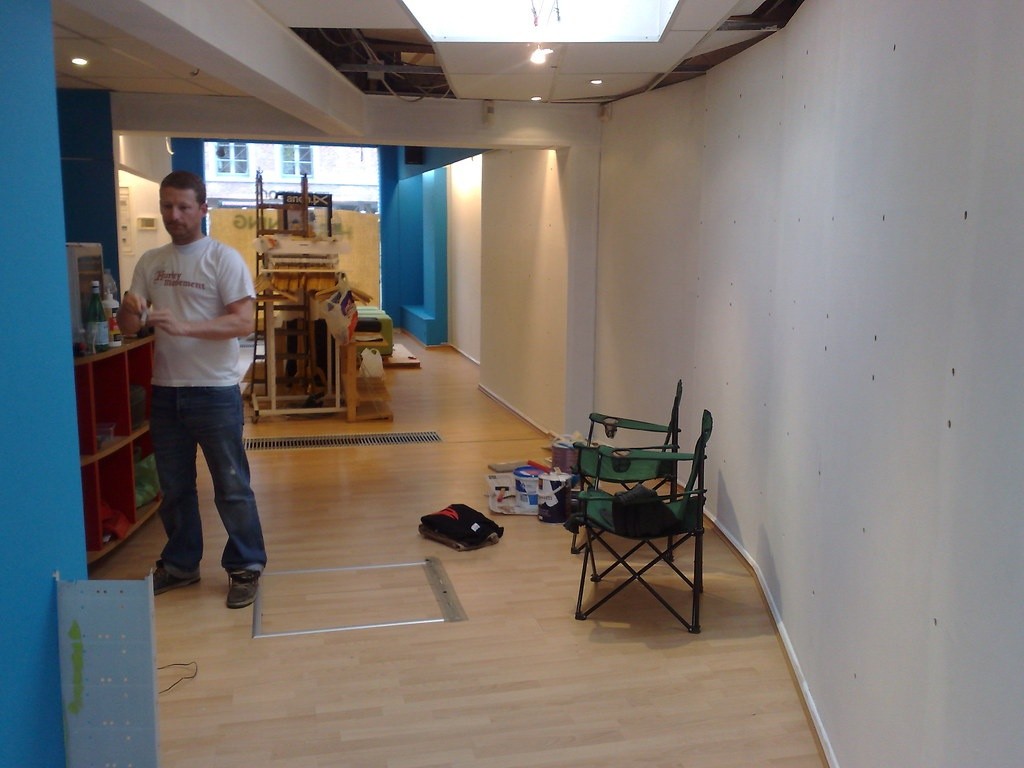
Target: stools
[356,306,393,358]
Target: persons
[117,170,267,608]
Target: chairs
[575,409,712,634]
[570,379,683,561]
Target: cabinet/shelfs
[73,329,165,566]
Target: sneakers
[226,568,258,609]
[153,558,200,595]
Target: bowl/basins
[97,423,115,445]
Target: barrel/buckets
[537,472,571,524]
[552,435,578,474]
[513,466,541,508]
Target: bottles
[86,281,110,352]
[103,268,117,300]
[101,294,122,346]
